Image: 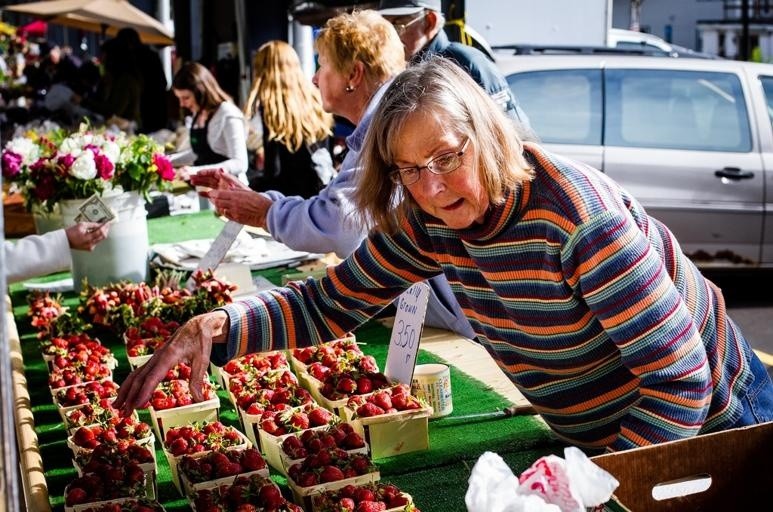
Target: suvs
[490,49,772,271]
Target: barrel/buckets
[60,189,151,293]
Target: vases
[32,188,155,295]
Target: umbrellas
[4,1,175,46]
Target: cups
[410,363,454,419]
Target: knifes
[442,405,538,421]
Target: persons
[382,1,530,141]
[189,11,481,355]
[0,26,173,137]
[166,62,253,193]
[108,57,771,457]
[240,38,342,199]
[3,218,108,283]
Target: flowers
[3,116,174,206]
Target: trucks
[292,0,718,166]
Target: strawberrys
[26,267,420,511]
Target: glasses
[385,136,472,187]
[393,12,428,37]
